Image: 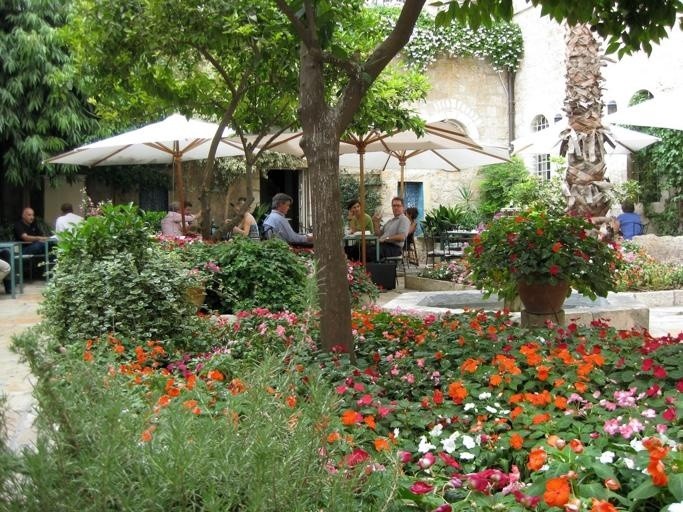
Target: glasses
[392,205,402,207]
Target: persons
[15,207,56,281]
[404,208,419,251]
[0,226,13,295]
[609,201,644,239]
[0,259,12,283]
[262,193,314,255]
[55,202,88,246]
[366,196,411,261]
[179,203,198,233]
[346,200,375,261]
[161,201,203,237]
[223,201,261,243]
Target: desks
[447,230,488,257]
[0,241,23,300]
[344,236,379,262]
[45,238,61,282]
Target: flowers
[160,233,223,286]
[460,209,628,309]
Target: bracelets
[347,218,352,222]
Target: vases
[183,286,206,314]
[516,273,572,315]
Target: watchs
[386,236,390,242]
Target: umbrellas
[42,113,272,234]
[219,120,485,267]
[603,87,683,131]
[510,115,664,154]
[340,145,515,197]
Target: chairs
[403,224,418,269]
[382,233,408,288]
[438,225,459,262]
[420,223,445,265]
[13,254,45,284]
[260,226,274,241]
[618,220,651,240]
